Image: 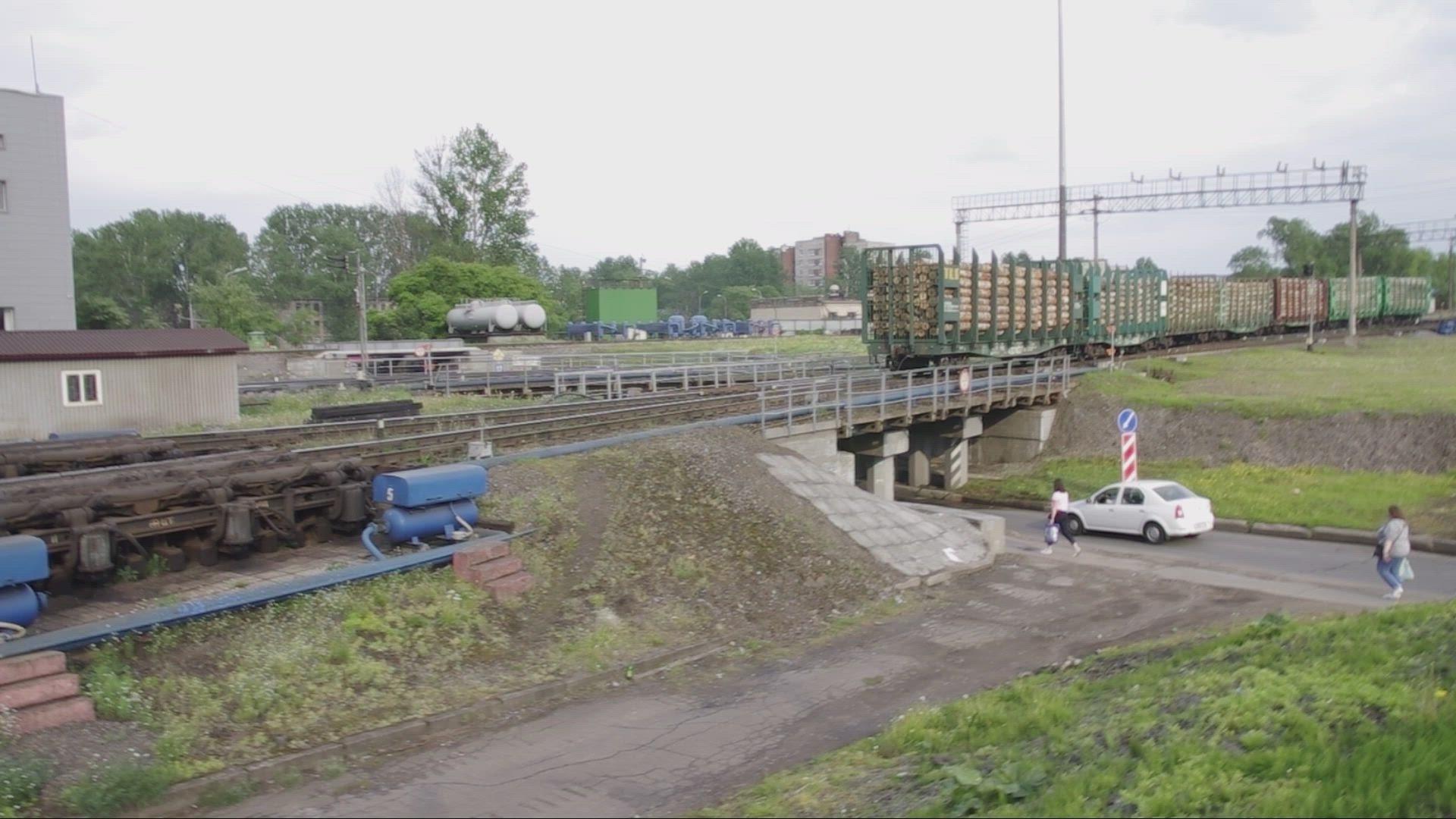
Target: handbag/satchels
[1043,521,1061,543]
[1398,557,1414,583]
[1374,544,1383,559]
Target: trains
[857,240,1436,375]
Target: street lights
[751,288,762,298]
[717,294,728,319]
[699,290,709,316]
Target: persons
[1106,497,1115,503]
[1376,505,1411,599]
[1040,478,1082,558]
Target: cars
[1066,479,1214,545]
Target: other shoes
[1381,592,1393,600]
[1072,542,1081,558]
[1392,587,1403,600]
[1038,545,1053,554]
